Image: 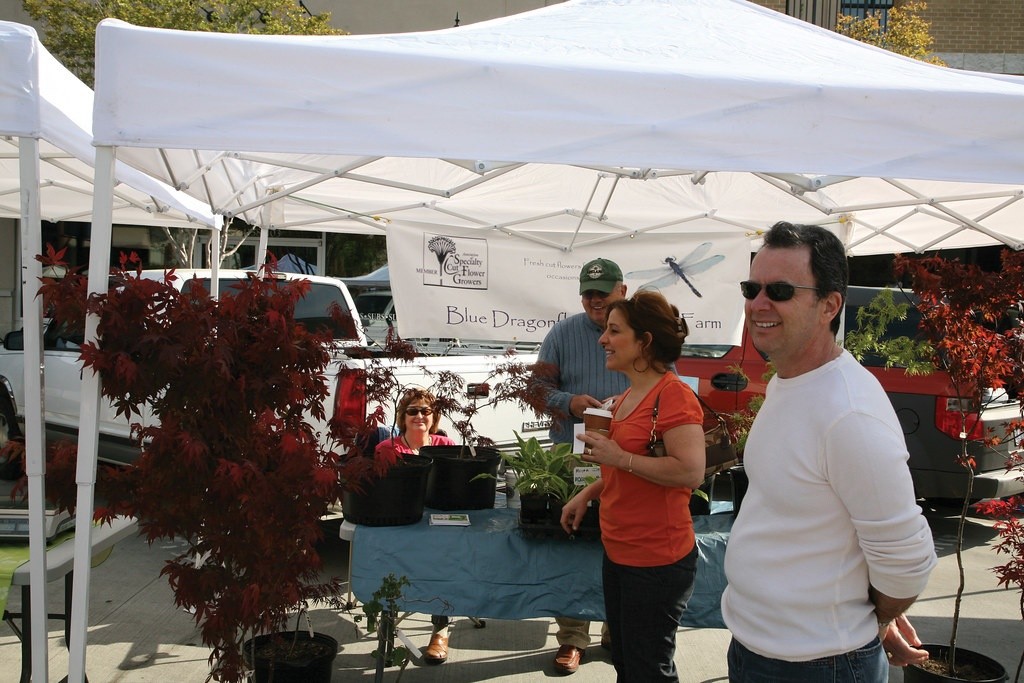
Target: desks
[337,509,736,683]
[0,513,139,683]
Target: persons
[526,258,680,674]
[373,387,456,665]
[720,220,938,683]
[559,289,708,683]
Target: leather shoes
[424,634,449,663]
[601,640,612,653]
[553,645,585,674]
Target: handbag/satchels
[648,381,738,480]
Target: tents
[0,0,1024,683]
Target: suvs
[676,284,1024,515]
[0,266,371,482]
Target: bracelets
[628,453,634,473]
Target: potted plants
[464,428,598,536]
[375,318,560,509]
[37,249,382,683]
[727,290,934,524]
[325,303,434,527]
[887,250,1024,683]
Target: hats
[579,258,623,295]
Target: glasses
[739,281,816,302]
[403,408,434,416]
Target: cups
[505,469,526,508]
[583,409,613,450]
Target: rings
[588,449,591,455]
